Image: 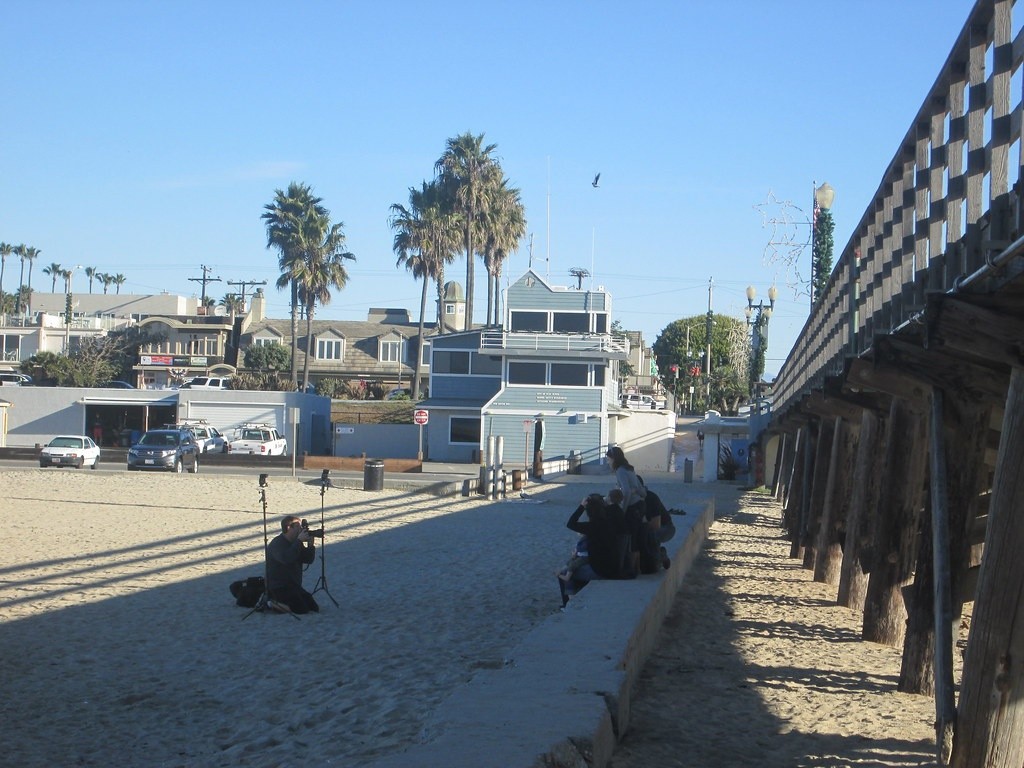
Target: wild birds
[519,489,532,501]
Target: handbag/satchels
[620,538,640,580]
[229,577,265,607]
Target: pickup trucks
[226,424,289,457]
[175,418,229,455]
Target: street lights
[66,266,84,349]
[745,283,777,412]
[684,321,717,366]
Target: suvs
[192,374,234,391]
[127,429,200,471]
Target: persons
[254,516,319,614]
[697,429,704,447]
[554,447,676,612]
[90,411,104,446]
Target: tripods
[310,469,338,610]
[241,474,302,623]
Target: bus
[0,374,35,387]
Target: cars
[389,388,413,400]
[177,381,192,390]
[107,380,137,389]
[296,380,316,394]
[40,435,101,468]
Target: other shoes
[661,547,671,569]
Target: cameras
[302,519,325,538]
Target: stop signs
[413,409,429,426]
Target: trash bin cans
[363,458,385,491]
[567,455,583,475]
